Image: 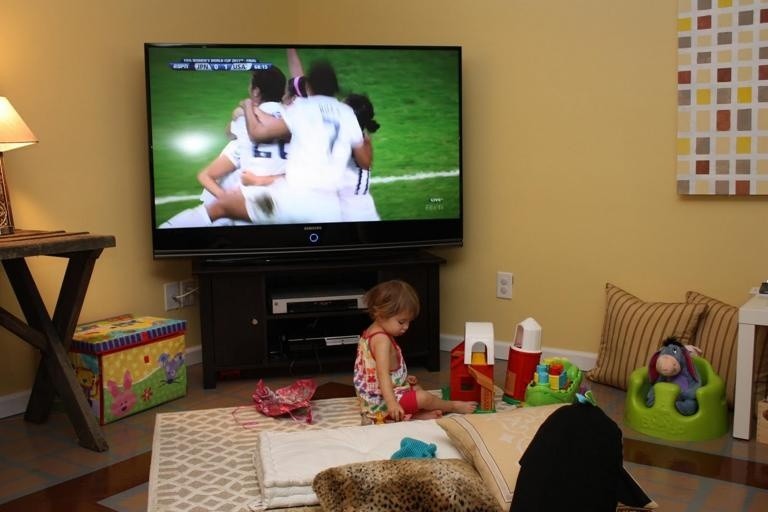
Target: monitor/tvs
[144,41,464,265]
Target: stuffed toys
[641,334,704,414]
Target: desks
[731,285,768,441]
[0,228,116,453]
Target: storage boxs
[756,397,768,446]
[69,313,187,426]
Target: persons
[159,48,381,227]
[352,279,479,423]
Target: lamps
[0,96,38,234]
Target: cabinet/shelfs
[193,251,449,390]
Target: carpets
[146,385,653,512]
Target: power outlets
[182,280,197,308]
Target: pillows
[311,458,502,512]
[586,281,709,392]
[436,401,661,511]
[685,291,766,420]
